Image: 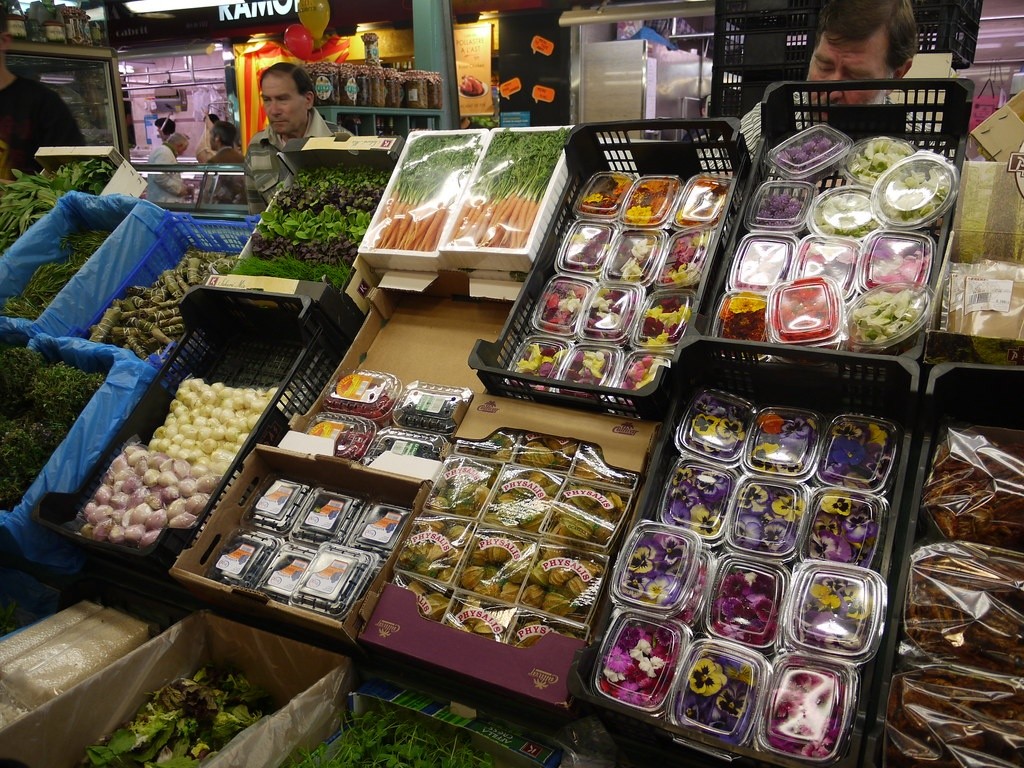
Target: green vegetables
[0,159,126,507]
[280,700,497,768]
[228,158,388,296]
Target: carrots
[373,128,571,252]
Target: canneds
[290,31,442,136]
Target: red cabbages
[88,665,266,768]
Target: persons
[0,3,81,181]
[682,0,917,171]
[204,121,248,208]
[148,131,190,205]
[155,117,176,142]
[242,62,354,209]
[195,113,235,162]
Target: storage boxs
[468,117,748,419]
[33,285,365,610]
[227,135,405,319]
[169,443,431,665]
[704,78,975,361]
[0,191,168,347]
[295,679,565,768]
[1,332,159,568]
[569,335,920,768]
[78,210,260,369]
[0,611,354,768]
[34,147,148,201]
[922,163,1024,366]
[864,362,1024,768]
